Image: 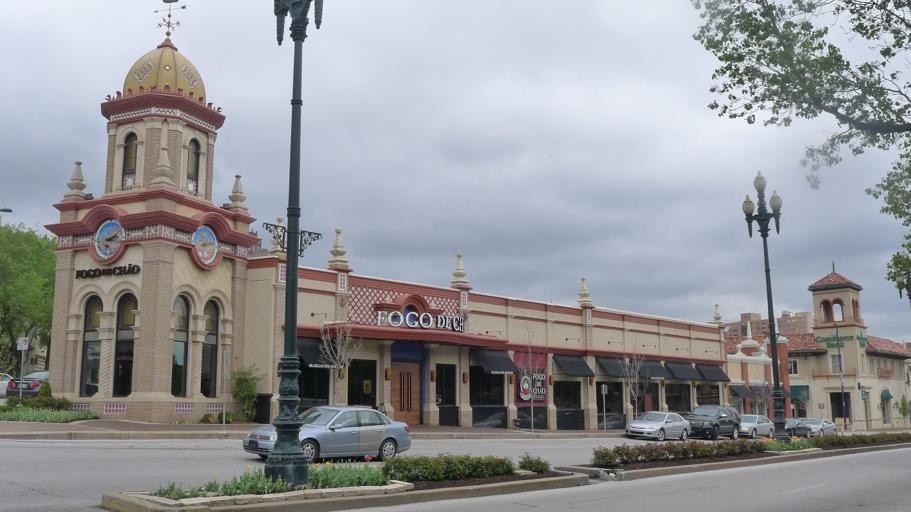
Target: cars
[0,370,49,400]
[473,410,538,429]
[784,416,838,439]
[600,413,626,430]
[738,413,777,439]
[241,404,412,465]
[625,410,692,442]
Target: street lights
[741,169,793,447]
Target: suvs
[685,404,742,441]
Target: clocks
[190,225,219,266]
[92,217,127,265]
